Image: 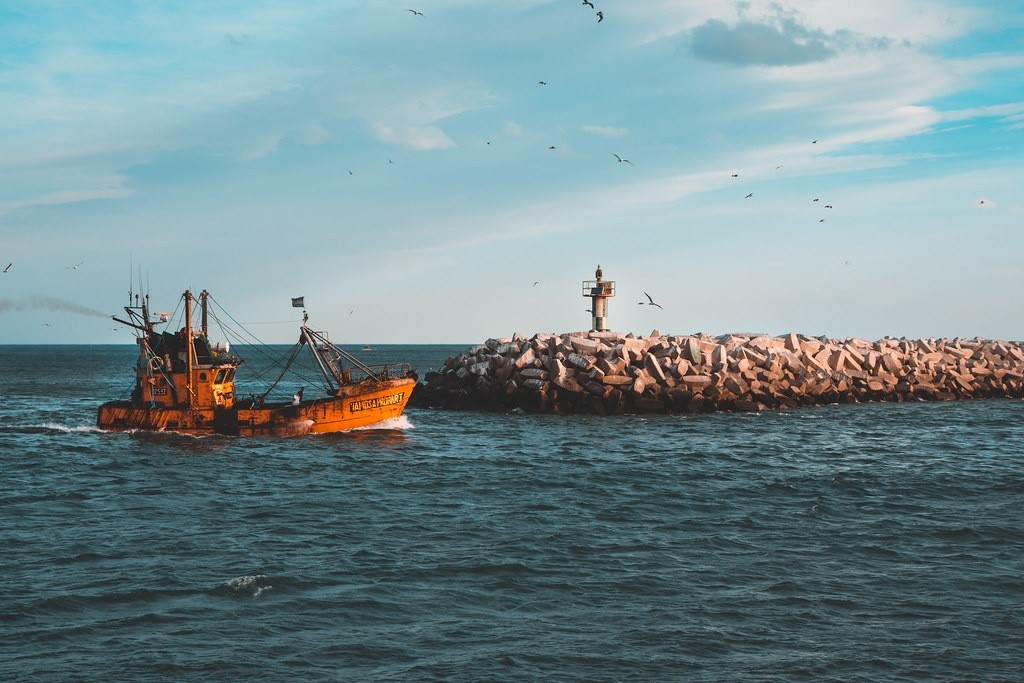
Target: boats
[96,286,416,435]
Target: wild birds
[583,0,594,9]
[3,261,123,333]
[348,82,984,287]
[597,12,604,23]
[409,10,423,16]
[638,292,663,310]
